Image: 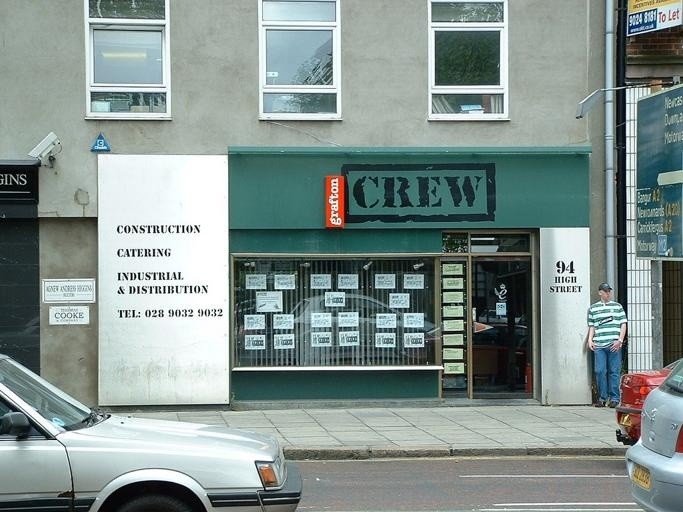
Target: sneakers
[595,401,608,407]
[609,401,619,408]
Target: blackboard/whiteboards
[637,83,683,261]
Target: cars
[614,360,682,446]
[409,320,528,384]
[0,353,303,510]
[279,291,434,356]
[624,361,682,512]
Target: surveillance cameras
[28,132,60,160]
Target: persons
[587,282,628,408]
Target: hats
[599,283,612,292]
